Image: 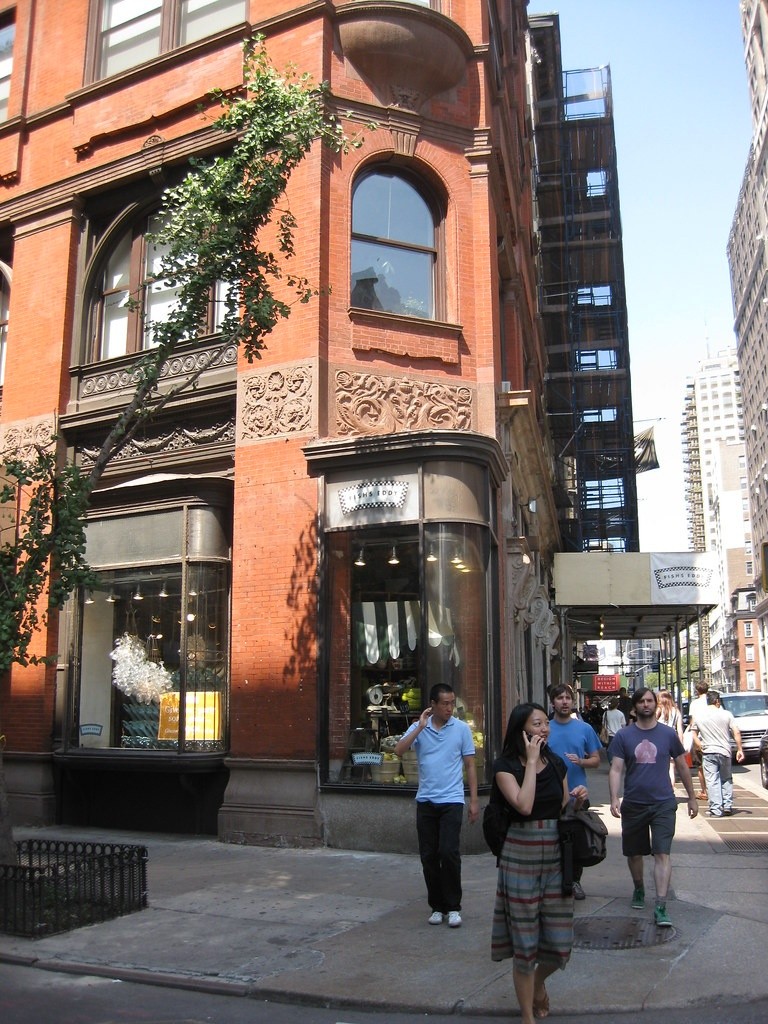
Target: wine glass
[110,629,173,705]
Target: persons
[394,683,480,927]
[608,688,699,926]
[578,680,744,818]
[491,683,604,1024]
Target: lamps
[83,584,95,607]
[157,580,169,600]
[132,581,145,602]
[386,545,402,567]
[423,541,440,563]
[449,552,475,576]
[104,584,118,605]
[354,547,367,568]
[185,579,201,599]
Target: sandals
[532,979,550,1019]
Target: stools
[334,724,384,787]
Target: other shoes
[705,811,720,817]
[724,811,731,816]
[700,794,708,799]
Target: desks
[367,713,422,757]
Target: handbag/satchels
[558,809,607,868]
[599,711,609,744]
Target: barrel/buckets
[370,760,401,783]
[401,752,421,783]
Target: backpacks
[482,754,564,857]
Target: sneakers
[428,910,443,925]
[631,887,645,908]
[447,910,462,927]
[572,881,584,900]
[654,903,673,926]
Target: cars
[677,692,768,790]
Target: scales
[365,677,417,712]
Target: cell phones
[522,733,545,751]
